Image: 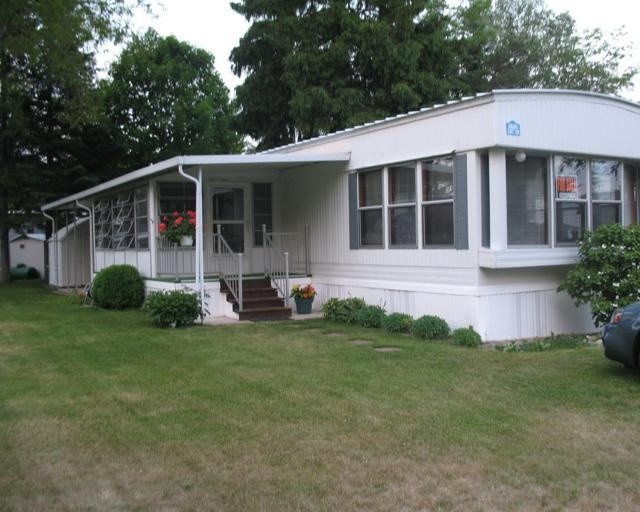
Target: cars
[602,299,640,375]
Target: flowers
[160,209,199,242]
[290,282,318,299]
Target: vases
[295,297,314,315]
[178,233,194,247]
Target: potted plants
[137,286,212,329]
